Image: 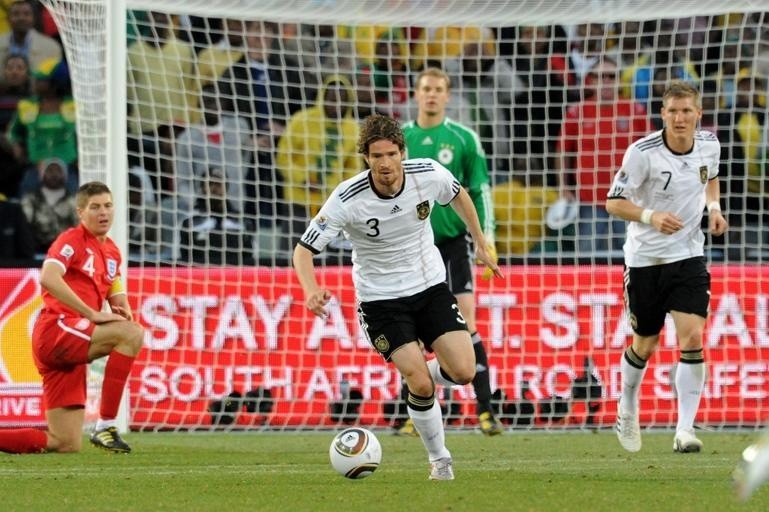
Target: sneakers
[672,435,702,453]
[430,458,454,481]
[394,418,420,436]
[89,427,129,452]
[478,412,503,435]
[615,398,641,452]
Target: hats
[26,58,68,80]
[39,158,68,185]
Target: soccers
[329,427,382,479]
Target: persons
[0,180,145,455]
[397,67,506,438]
[0,1,768,266]
[289,114,507,482]
[603,82,729,455]
